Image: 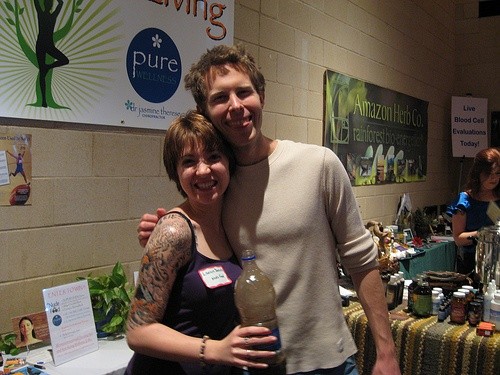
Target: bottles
[408,276,500,332]
[234,250,286,375]
[380,269,406,308]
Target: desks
[343,236,500,375]
[18,333,134,375]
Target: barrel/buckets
[467,220,500,290]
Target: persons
[15,316,44,348]
[6,150,28,183]
[137,45,401,375]
[123,110,277,375]
[446,147,500,274]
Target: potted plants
[77,262,131,337]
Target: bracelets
[198,335,209,362]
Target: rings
[246,349,250,357]
[244,337,249,347]
